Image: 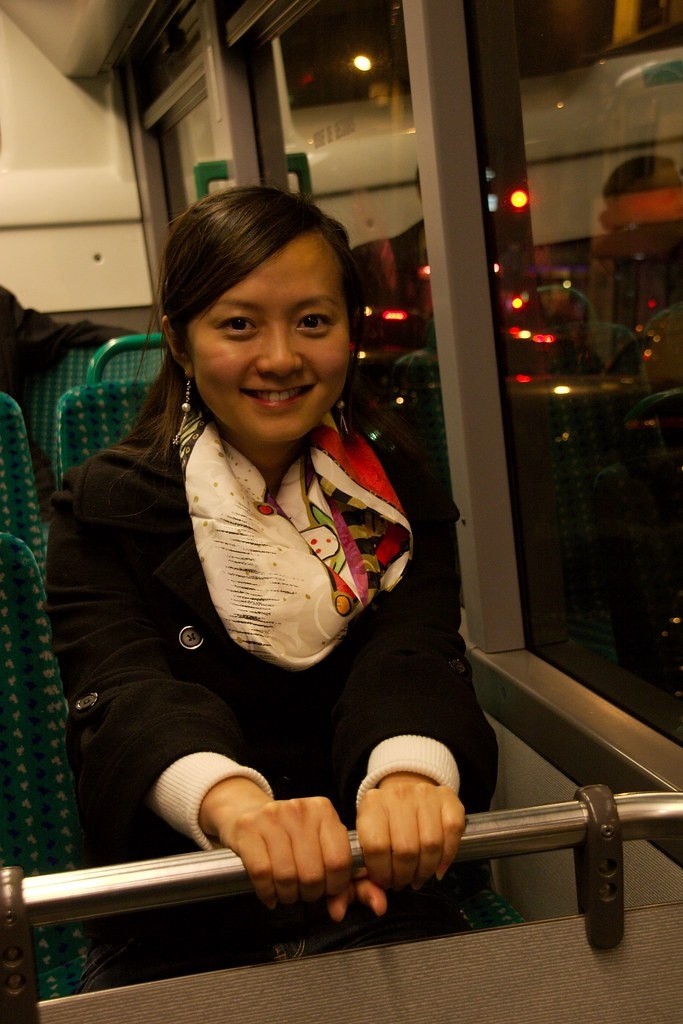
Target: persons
[44,179,465,921]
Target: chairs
[1,332,523,999]
[383,285,683,694]
